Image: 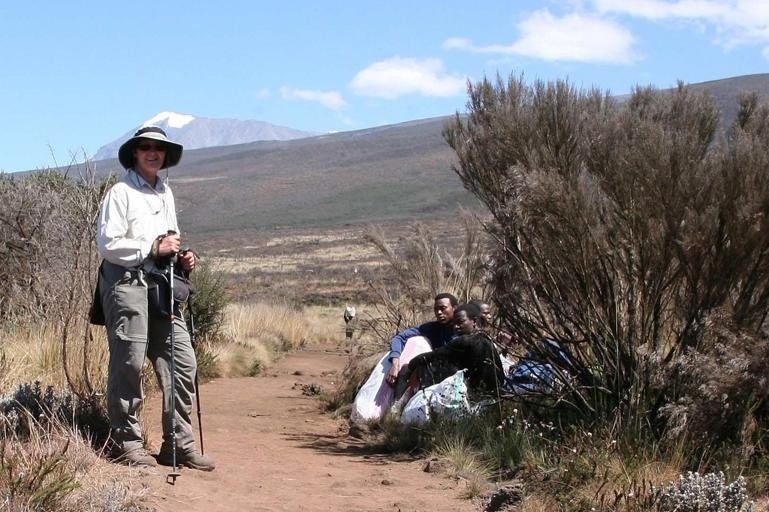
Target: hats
[119,124,183,170]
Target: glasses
[135,142,166,152]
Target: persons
[343,301,355,354]
[382,291,519,426]
[95,125,216,473]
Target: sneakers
[158,447,215,471]
[110,444,158,466]
